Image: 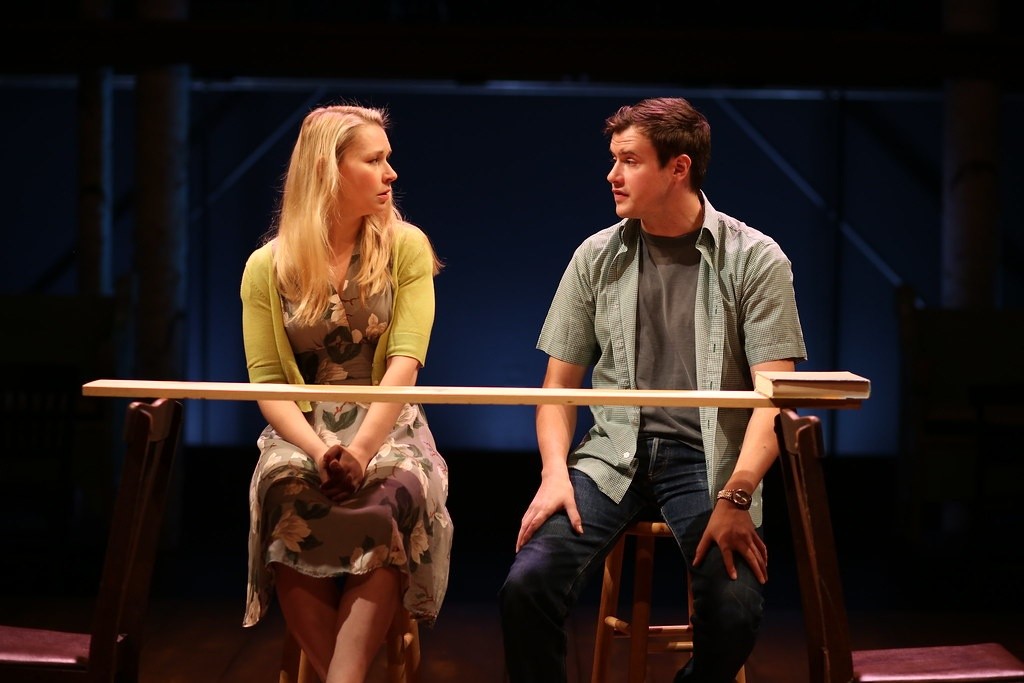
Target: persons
[497,98,808,683]
[240,106,454,683]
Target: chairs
[776,404,1024,683]
[1,397,178,682]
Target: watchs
[717,488,752,511]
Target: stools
[280,587,408,683]
[587,514,747,683]
